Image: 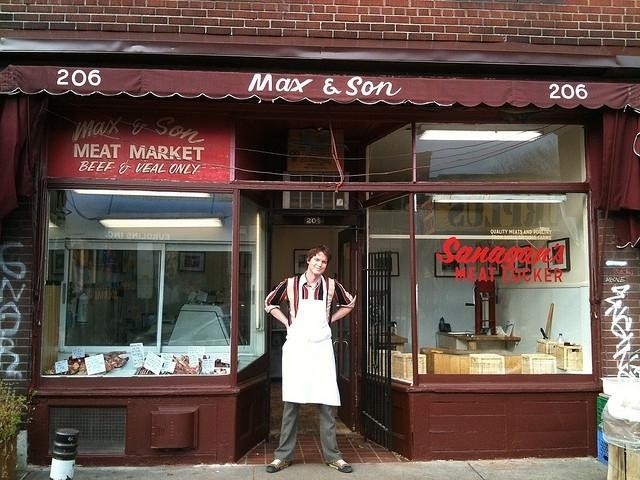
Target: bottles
[556,333,564,345]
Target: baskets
[537,340,582,371]
[597,396,609,464]
[377,350,556,383]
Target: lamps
[417,123,543,143]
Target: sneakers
[266,458,291,473]
[329,460,352,472]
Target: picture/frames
[293,248,308,276]
[178,251,205,272]
[369,237,572,278]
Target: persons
[263,244,356,473]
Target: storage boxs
[375,338,582,383]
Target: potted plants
[0,379,38,479]
